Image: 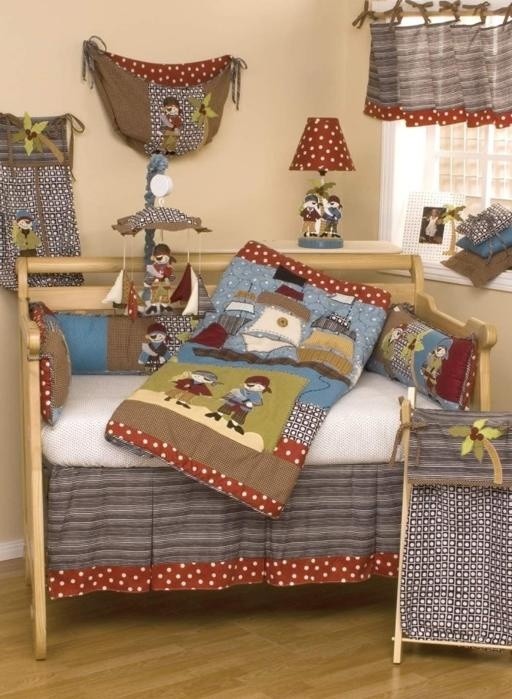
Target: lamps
[288,112,356,247]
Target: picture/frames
[400,188,464,262]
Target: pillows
[28,298,205,428]
[365,295,476,403]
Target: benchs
[16,245,497,657]
